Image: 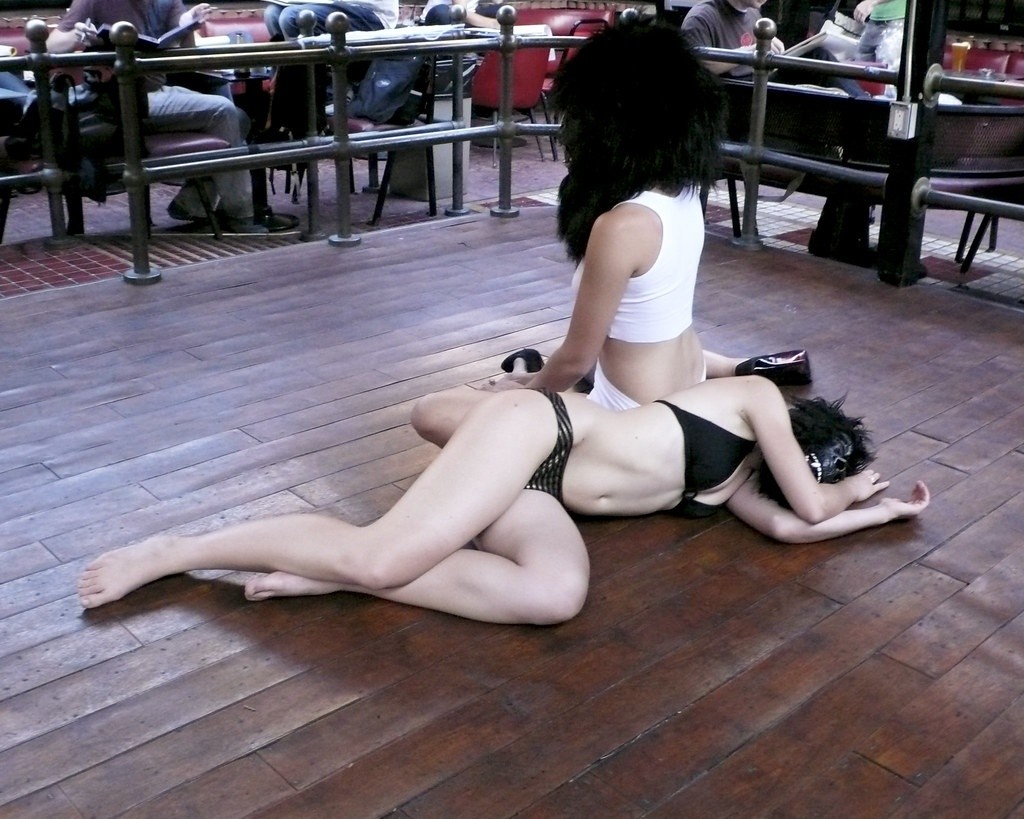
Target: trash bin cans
[387,53,481,201]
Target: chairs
[471,34,552,168]
[543,20,609,161]
[97,39,230,237]
[325,6,452,225]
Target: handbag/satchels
[19,71,84,173]
[347,55,427,125]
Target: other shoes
[166,199,210,222]
[214,206,270,239]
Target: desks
[196,64,299,231]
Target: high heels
[736,348,813,386]
[502,349,545,373]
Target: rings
[867,476,875,483]
[489,378,495,385]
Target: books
[93,20,199,51]
[769,31,827,75]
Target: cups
[952,43,967,72]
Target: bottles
[234,33,251,78]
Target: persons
[45,0,269,237]
[77,375,930,626]
[853,0,907,101]
[682,0,875,267]
[264,0,399,116]
[409,23,813,448]
